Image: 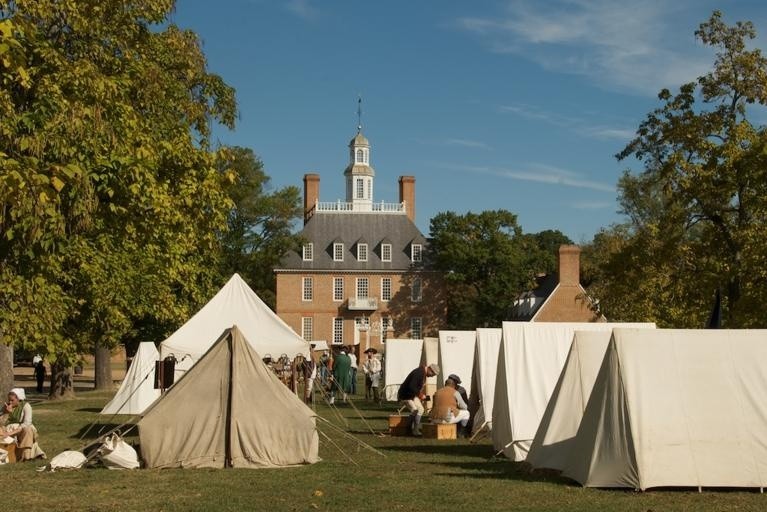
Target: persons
[319,345,382,405]
[0,353,47,463]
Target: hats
[363,347,378,354]
[430,364,440,376]
[449,374,462,383]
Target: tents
[384,321,660,471]
[158,272,317,397]
[560,327,766,493]
[129,325,323,470]
[99,341,161,416]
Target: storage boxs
[422,424,458,440]
[390,415,411,432]
[0,443,16,463]
